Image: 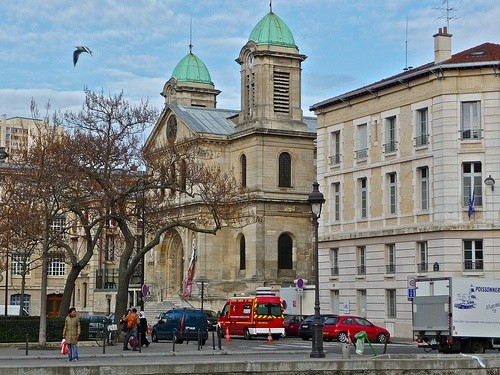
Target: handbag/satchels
[61,338,69,354]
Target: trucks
[216,294,284,340]
[409,271,500,354]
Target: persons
[120,308,150,351]
[63,307,80,362]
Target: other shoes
[65,359,79,363]
[146,342,150,347]
[123,347,138,351]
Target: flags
[468,192,475,214]
[184,248,196,297]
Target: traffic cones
[225,325,231,342]
[267,328,273,345]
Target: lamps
[484,175,495,191]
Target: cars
[283,314,309,337]
[196,310,217,331]
[80,315,107,338]
[297,314,339,341]
[107,313,115,323]
[322,316,390,344]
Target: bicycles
[95,331,119,347]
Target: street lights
[106,294,111,316]
[306,181,327,358]
[135,178,150,348]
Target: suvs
[152,307,209,346]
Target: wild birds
[73,45,95,68]
